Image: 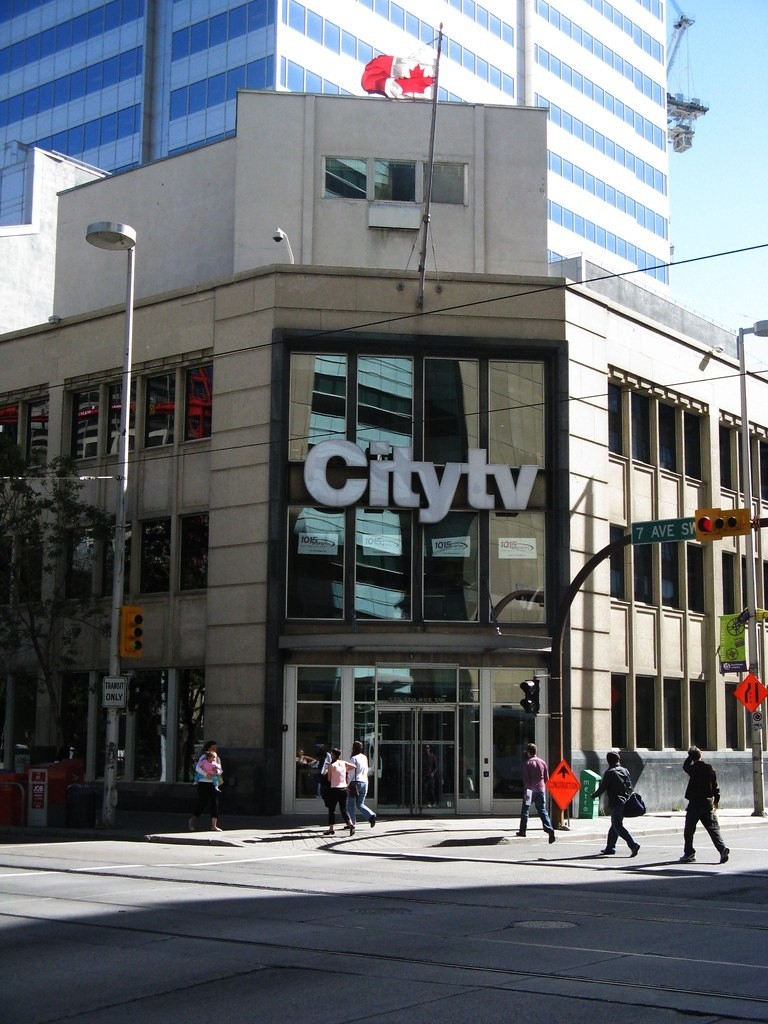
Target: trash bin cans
[66,784,98,829]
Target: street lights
[84,219,137,833]
[736,320,767,818]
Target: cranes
[664,15,709,154]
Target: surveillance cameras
[273,229,284,242]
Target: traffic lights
[520,679,539,713]
[694,508,752,540]
[120,606,144,660]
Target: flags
[361,55,439,99]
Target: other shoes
[631,845,640,857]
[549,830,555,843]
[601,849,615,853]
[680,857,695,862]
[344,826,349,829]
[370,815,376,828]
[720,848,730,863]
[516,832,526,836]
[188,818,194,831]
[350,828,355,836]
[211,827,222,832]
[324,830,334,835]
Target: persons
[678,746,729,863]
[591,752,640,857]
[464,769,475,796]
[517,744,554,844]
[344,741,375,828]
[324,748,357,836]
[296,744,331,798]
[423,744,438,807]
[188,741,223,831]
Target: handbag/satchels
[348,782,360,797]
[623,792,645,817]
